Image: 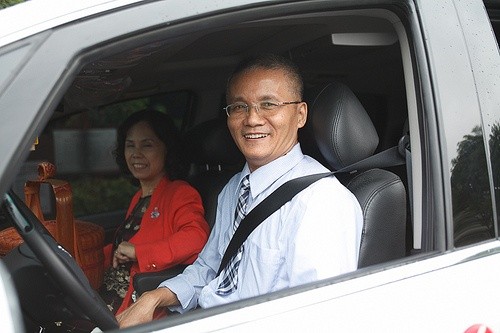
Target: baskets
[0,162,105,289]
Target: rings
[114,251,119,256]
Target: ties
[216,175,250,296]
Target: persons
[91,55,366,333]
[25,109,211,333]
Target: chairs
[297,81,407,269]
[133,118,241,295]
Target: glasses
[222,99,302,118]
